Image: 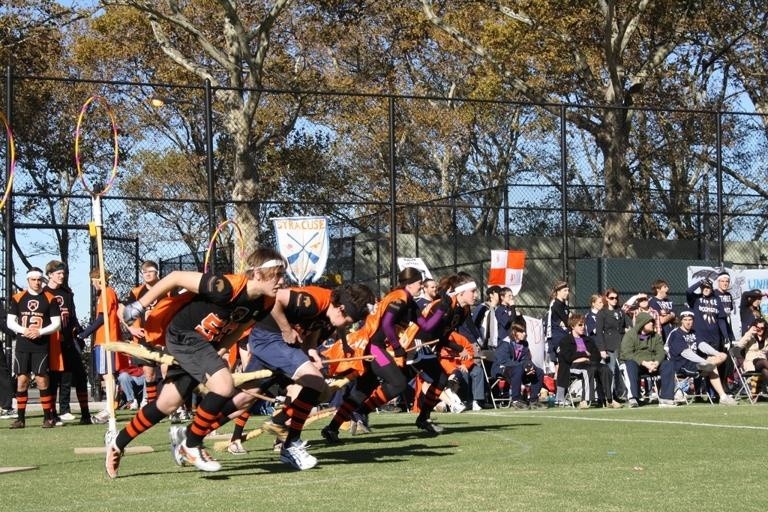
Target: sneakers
[321,425,345,446]
[659,399,680,405]
[57,412,76,420]
[130,398,138,410]
[472,401,481,411]
[511,398,640,409]
[696,361,716,372]
[140,401,147,409]
[449,401,467,414]
[1,409,18,418]
[719,395,740,405]
[8,419,23,429]
[42,418,65,429]
[80,414,105,425]
[169,406,318,473]
[416,417,444,433]
[353,409,372,431]
[104,431,123,477]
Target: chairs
[727,340,768,404]
[548,356,715,410]
[475,349,514,409]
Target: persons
[7,268,58,428]
[0,296,17,418]
[472,271,768,413]
[76,249,485,478]
[44,259,93,426]
[60,384,73,421]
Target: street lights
[147,96,225,119]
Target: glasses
[607,296,619,300]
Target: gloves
[700,279,713,287]
[724,338,730,351]
[122,301,143,327]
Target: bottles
[547,392,556,409]
